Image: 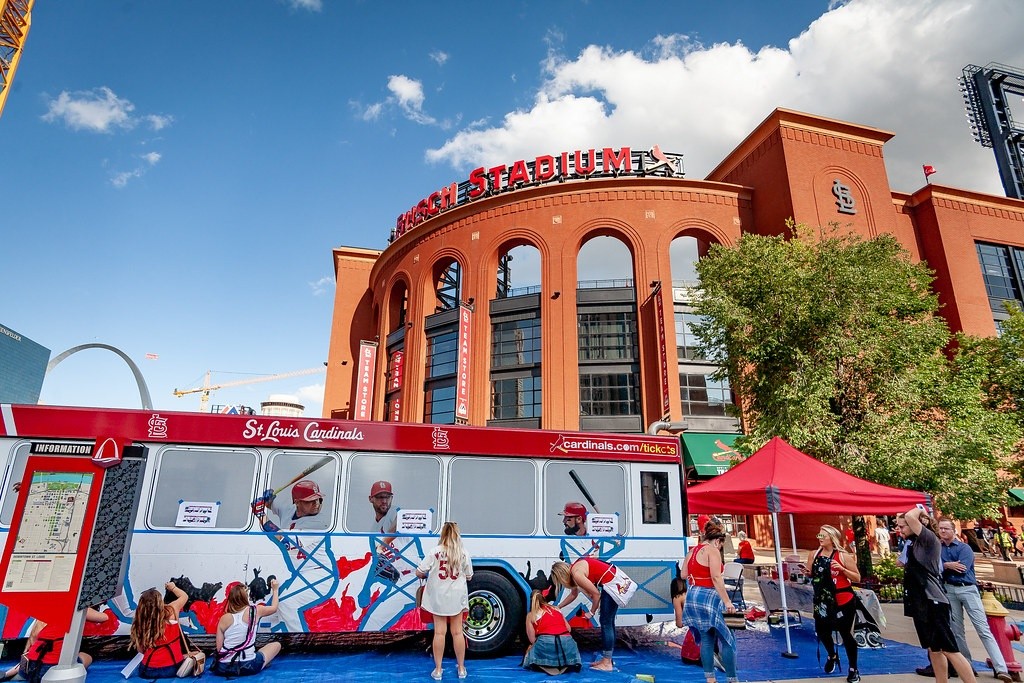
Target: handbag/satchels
[1016,538,1024,553]
[603,565,638,608]
[177,653,206,677]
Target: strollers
[830,588,887,648]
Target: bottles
[798,574,803,584]
[791,568,795,577]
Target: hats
[371,481,394,499]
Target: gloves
[250,498,264,516]
[264,490,276,506]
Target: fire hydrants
[980,582,1024,682]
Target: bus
[0,403,693,662]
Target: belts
[943,579,973,587]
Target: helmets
[557,502,587,521]
[291,480,327,504]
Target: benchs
[743,563,775,581]
[991,561,1024,584]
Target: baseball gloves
[374,553,400,582]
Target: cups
[772,571,778,579]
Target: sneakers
[846,668,859,683]
[825,653,839,673]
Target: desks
[756,577,888,649]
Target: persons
[670,517,739,683]
[416,521,473,680]
[264,480,329,529]
[0,607,108,683]
[131,582,199,678]
[974,521,1024,561]
[522,589,582,675]
[551,557,618,671]
[368,481,401,532]
[875,506,1012,683]
[734,530,755,564]
[216,579,281,676]
[800,524,861,683]
[558,501,589,535]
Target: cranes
[172,362,328,413]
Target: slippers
[430,667,443,680]
[458,665,467,679]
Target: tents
[687,436,932,653]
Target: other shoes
[590,663,613,671]
[0,669,18,682]
[1021,551,1023,556]
[916,664,939,677]
[997,671,1013,683]
[590,660,615,666]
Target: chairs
[721,562,747,610]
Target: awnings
[1008,489,1024,501]
[681,433,747,475]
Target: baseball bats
[273,456,334,496]
[569,468,599,512]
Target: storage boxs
[783,562,804,580]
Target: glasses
[141,587,156,595]
[898,523,909,529]
[816,534,829,539]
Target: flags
[923,166,936,177]
